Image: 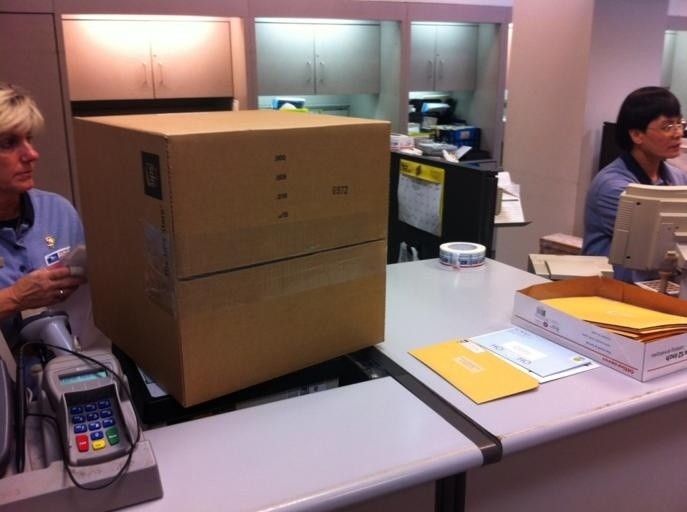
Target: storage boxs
[72,109,391,408]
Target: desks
[101,257,687,512]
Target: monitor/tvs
[607,183,687,299]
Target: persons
[583,86,687,287]
[0,80,92,395]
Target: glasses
[645,121,687,134]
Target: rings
[59,289,65,299]
